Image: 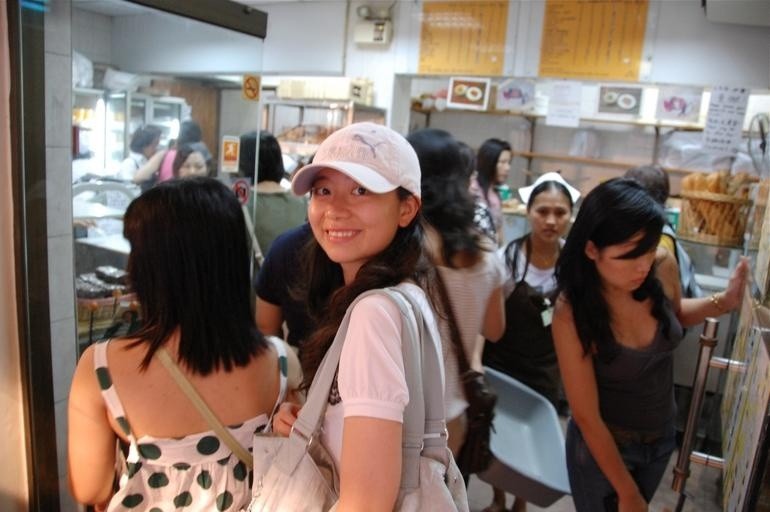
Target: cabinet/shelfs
[412,95,702,177]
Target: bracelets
[711,294,730,316]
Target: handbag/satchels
[244,287,468,512]
[422,251,497,481]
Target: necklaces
[531,243,560,267]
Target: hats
[290,122,423,203]
[518,171,582,202]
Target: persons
[552,176,746,512]
[171,141,216,181]
[254,148,344,347]
[468,138,513,249]
[66,174,307,512]
[622,164,702,340]
[229,130,309,340]
[479,171,583,512]
[113,124,161,181]
[248,122,470,512]
[132,121,201,189]
[396,128,506,490]
